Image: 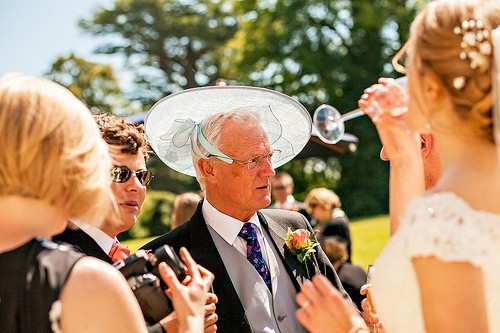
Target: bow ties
[108,241,130,262]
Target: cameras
[113,245,188,326]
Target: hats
[145,85,313,177]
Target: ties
[238,223,272,294]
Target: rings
[301,300,310,310]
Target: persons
[51,111,218,332]
[169,171,368,318]
[138,85,369,333]
[294,0,500,333]
[360,128,443,332]
[0,74,216,333]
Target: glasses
[110,166,155,186]
[207,150,283,169]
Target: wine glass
[313,77,409,144]
[367,266,383,333]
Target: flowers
[286,226,321,278]
